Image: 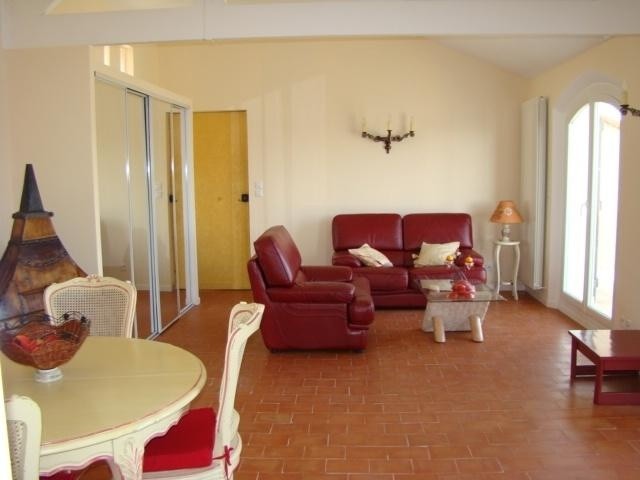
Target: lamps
[489,201,524,242]
[361,112,414,154]
[619,80,640,117]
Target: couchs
[332,212,487,307]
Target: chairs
[142,301,265,479]
[246,225,374,352]
[5,393,92,480]
[43,274,137,339]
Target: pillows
[414,241,461,268]
[348,243,393,269]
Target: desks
[568,329,640,405]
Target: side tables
[492,240,520,301]
